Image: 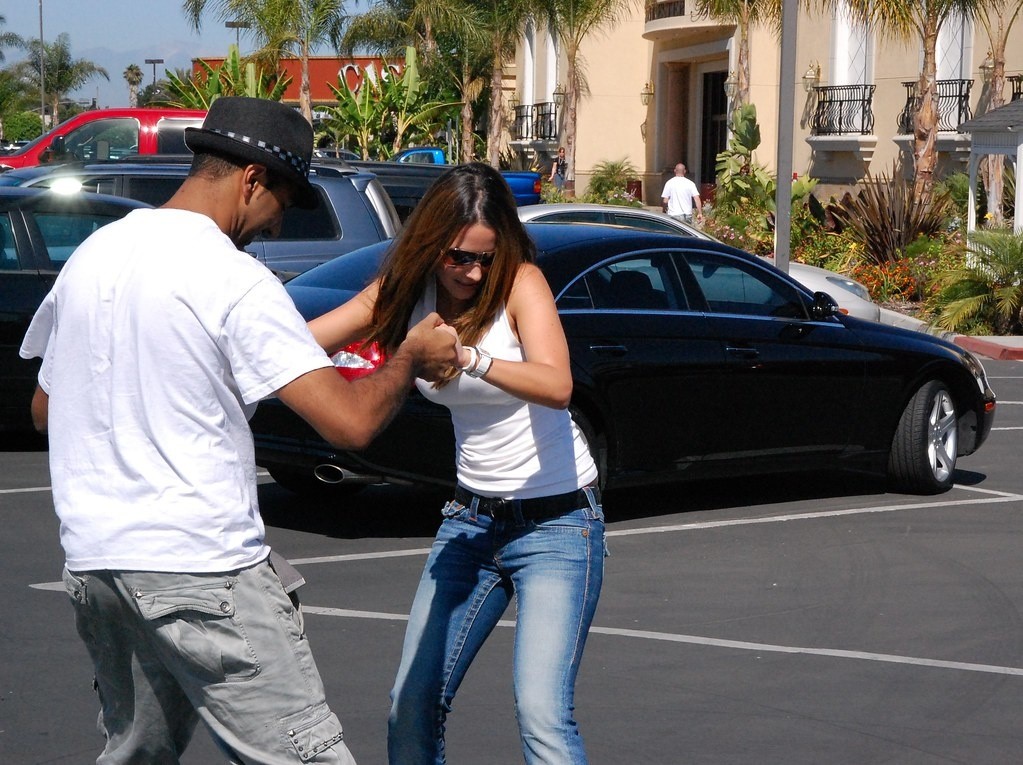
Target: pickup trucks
[0,107,206,175]
[383,146,542,206]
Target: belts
[454,483,601,524]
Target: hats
[185,97,320,210]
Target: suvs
[0,157,403,274]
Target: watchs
[467,345,493,380]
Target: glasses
[441,248,496,267]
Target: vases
[699,183,717,210]
[618,181,642,203]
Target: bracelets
[460,345,476,373]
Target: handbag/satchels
[684,214,692,224]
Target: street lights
[144,58,165,83]
[224,21,252,50]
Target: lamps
[803,59,823,94]
[552,82,568,108]
[639,80,654,106]
[507,91,520,112]
[979,46,996,85]
[723,69,740,100]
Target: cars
[0,187,301,407]
[514,202,884,323]
[308,146,362,161]
[248,224,1001,514]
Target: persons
[304,161,609,765]
[19,96,457,764]
[661,162,702,226]
[547,147,567,193]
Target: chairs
[89,139,110,161]
[604,272,666,308]
